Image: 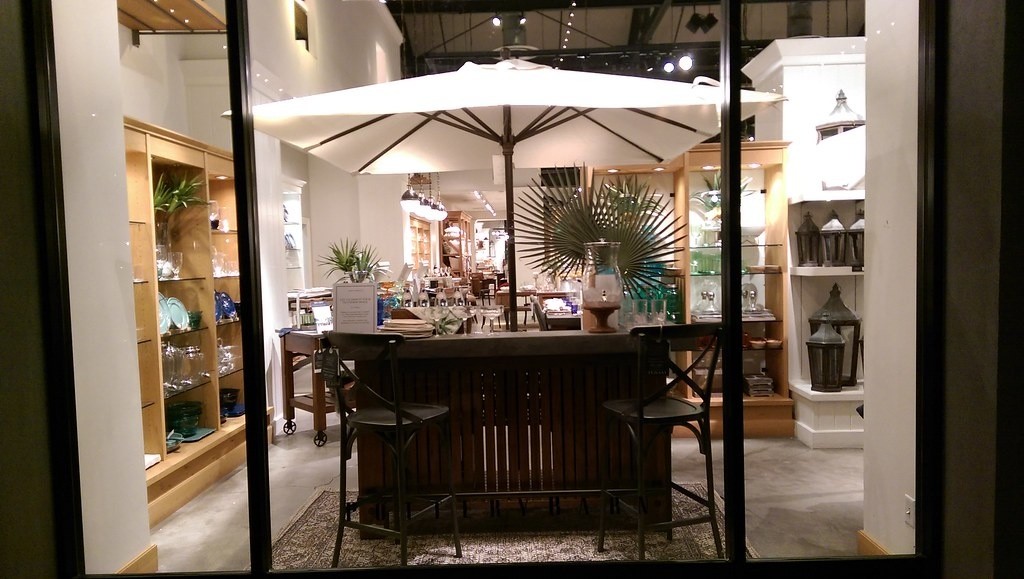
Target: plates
[376,319,435,337]
[158,293,190,334]
[214,290,236,323]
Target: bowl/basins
[166,432,183,452]
[165,400,207,438]
[219,388,240,412]
[500,287,509,292]
[750,340,782,349]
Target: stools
[323,328,467,568]
[595,319,731,560]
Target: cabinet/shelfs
[125,119,247,479]
[781,187,874,401]
[407,216,433,268]
[679,134,784,409]
[438,210,472,278]
[281,174,306,290]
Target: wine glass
[209,200,220,230]
[690,224,702,247]
[425,307,450,338]
[157,245,183,281]
[448,306,477,336]
[212,253,239,277]
[440,266,451,278]
[381,282,394,296]
[479,305,505,336]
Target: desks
[338,325,679,537]
[501,285,538,329]
[278,329,335,446]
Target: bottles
[218,345,236,374]
[697,289,762,314]
[623,282,681,322]
[405,290,411,307]
[161,341,204,399]
[455,288,464,306]
[420,289,428,307]
[438,288,447,307]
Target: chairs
[481,289,500,327]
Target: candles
[593,270,619,301]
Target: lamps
[518,15,526,25]
[399,168,449,220]
[686,5,720,36]
[642,57,654,72]
[492,16,503,26]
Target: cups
[563,299,569,303]
[632,299,647,325]
[566,302,572,307]
[652,300,666,325]
[571,305,578,315]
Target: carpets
[246,478,765,571]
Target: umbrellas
[222,57,787,334]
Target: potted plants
[689,168,758,222]
